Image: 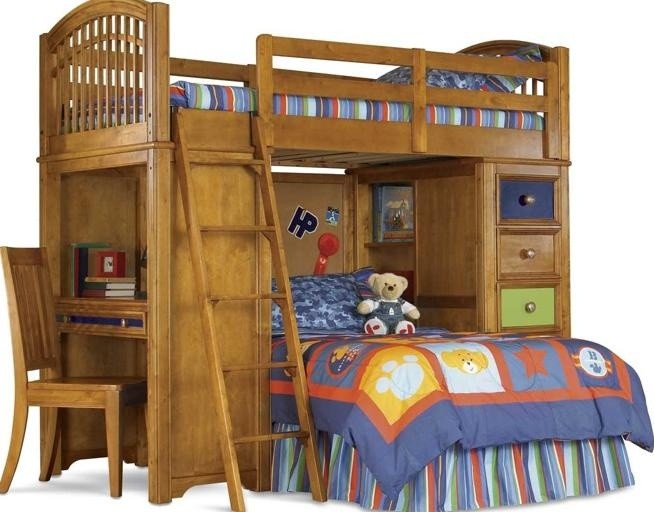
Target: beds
[37,0,654,512]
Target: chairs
[0,242,144,498]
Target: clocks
[91,249,127,280]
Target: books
[69,243,137,300]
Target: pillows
[372,41,544,98]
[268,271,363,337]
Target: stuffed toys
[357,273,422,334]
